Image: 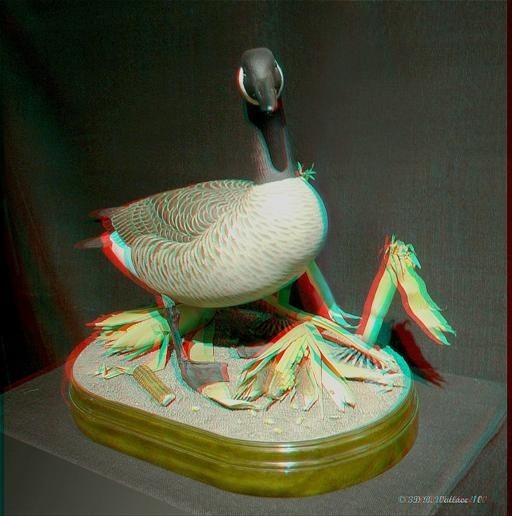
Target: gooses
[71,47,364,411]
